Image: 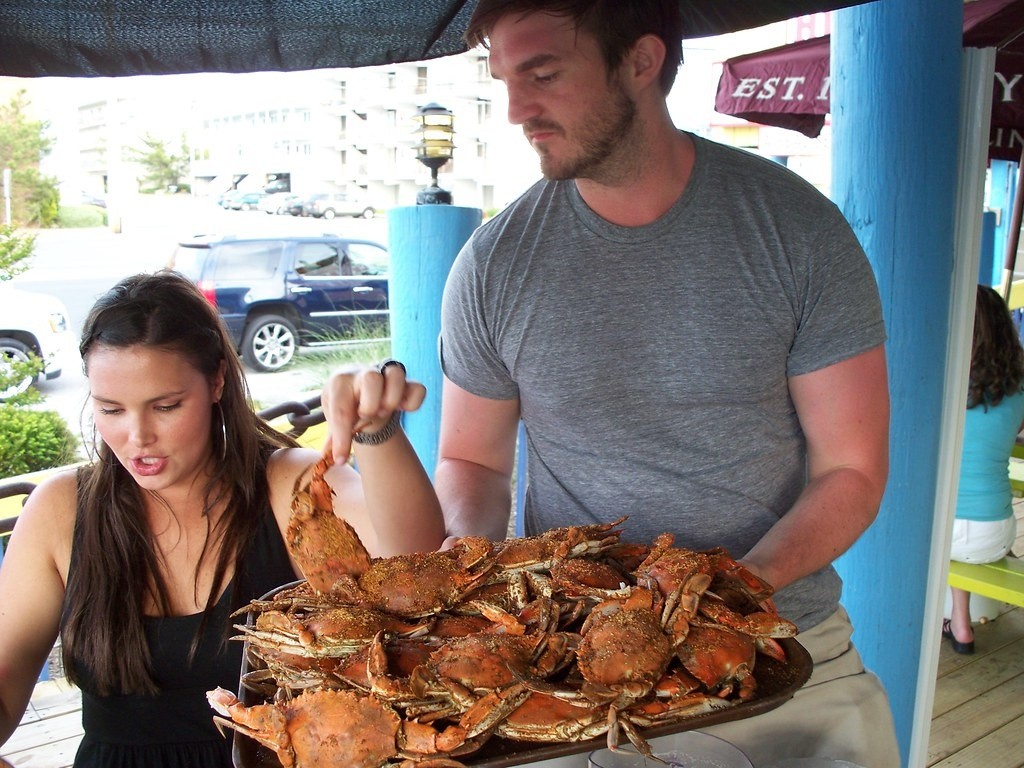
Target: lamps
[410,101,457,206]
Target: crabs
[205,421,800,768]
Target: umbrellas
[716,1,1024,307]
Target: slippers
[942,618,975,655]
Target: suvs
[163,228,388,374]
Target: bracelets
[353,409,403,446]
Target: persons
[0,274,445,768]
[434,1,901,768]
[942,285,1024,653]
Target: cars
[0,298,66,401]
[217,190,377,219]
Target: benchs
[946,557,1024,611]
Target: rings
[377,358,406,378]
